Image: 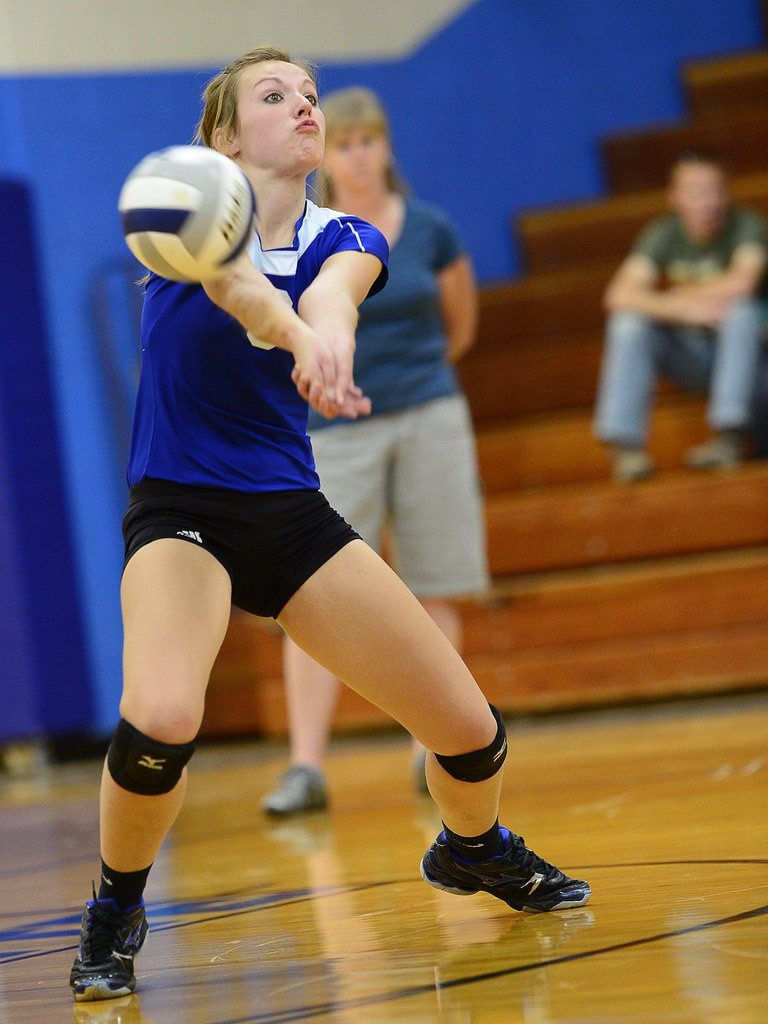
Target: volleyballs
[115,142,261,282]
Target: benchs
[199,47,768,737]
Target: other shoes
[685,437,740,471]
[614,450,649,485]
[261,764,326,817]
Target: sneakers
[420,830,591,912]
[68,879,149,1002]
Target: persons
[591,150,767,484]
[71,42,591,999]
[252,88,501,818]
